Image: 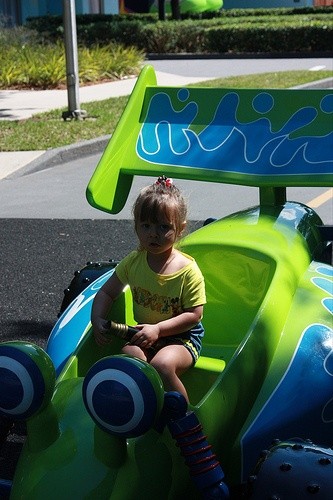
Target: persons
[88,174,208,408]
